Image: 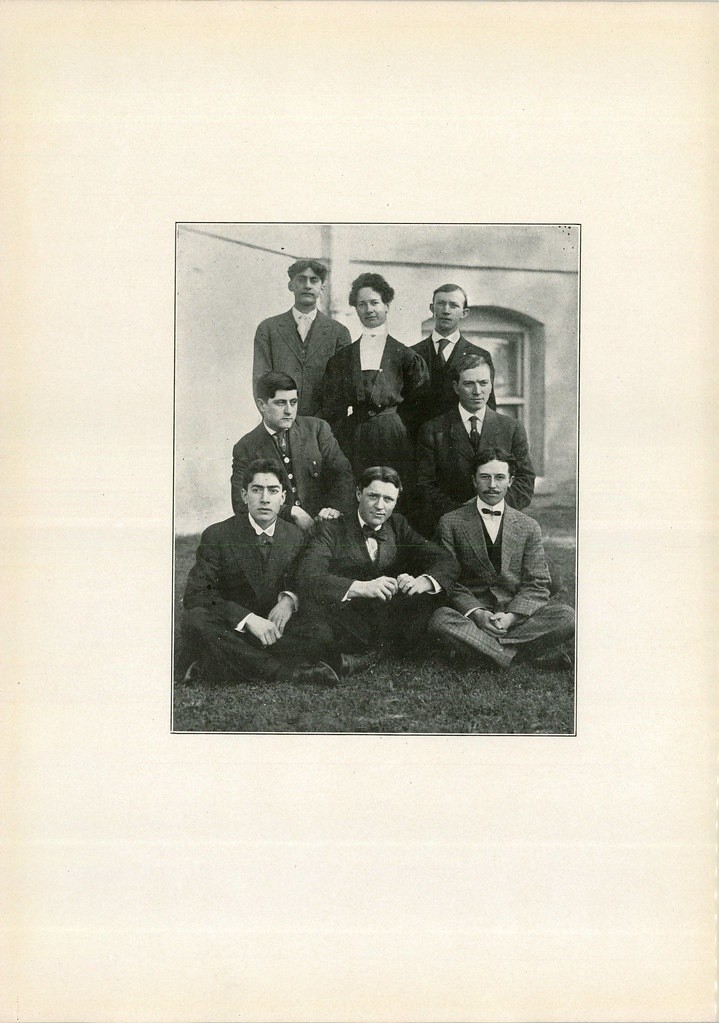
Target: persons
[415,355,536,538]
[252,260,353,417]
[293,466,461,677]
[322,273,432,479]
[182,458,341,687]
[409,284,497,412]
[229,371,357,535]
[427,447,574,669]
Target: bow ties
[362,524,387,544]
[481,508,502,517]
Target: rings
[328,513,334,517]
[408,583,412,587]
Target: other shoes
[183,657,215,686]
[337,652,362,678]
[531,647,573,671]
[301,659,339,685]
[451,647,467,661]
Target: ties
[436,339,450,370]
[468,416,480,455]
[274,430,284,451]
